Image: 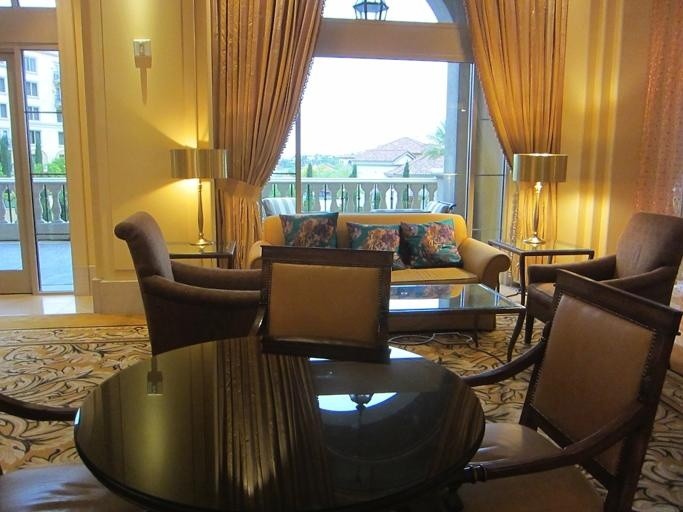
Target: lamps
[351,0,391,23]
[167,145,230,248]
[143,355,167,399]
[509,151,571,246]
[129,36,155,110]
[347,392,374,426]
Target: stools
[0,388,148,511]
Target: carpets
[0,312,683,511]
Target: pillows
[343,218,408,271]
[397,215,464,269]
[277,211,340,249]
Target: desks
[484,236,594,306]
[164,240,241,271]
[65,334,488,511]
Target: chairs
[442,262,683,511]
[425,198,456,214]
[108,207,267,356]
[524,210,683,344]
[260,194,296,216]
[257,243,393,349]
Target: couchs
[244,211,513,334]
